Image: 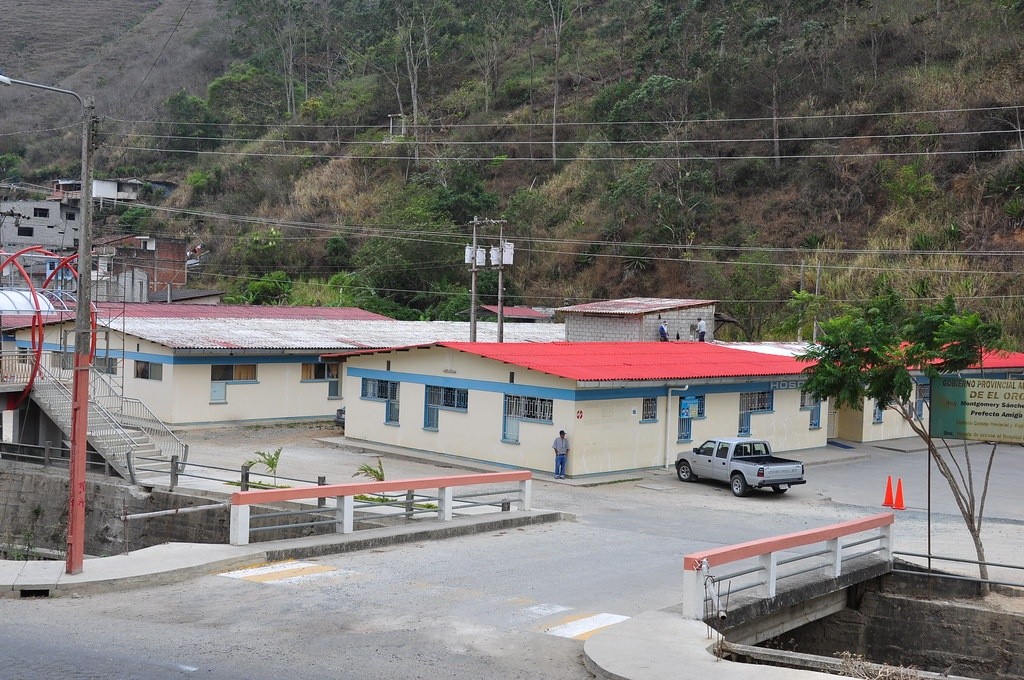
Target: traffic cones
[891,478,907,510]
[881,475,895,506]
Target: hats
[560,431,566,435]
[663,321,668,324]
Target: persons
[552,430,570,479]
[659,321,669,342]
[696,317,706,342]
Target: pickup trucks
[674,438,807,498]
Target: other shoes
[555,476,565,479]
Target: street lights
[0,73,94,575]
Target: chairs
[717,447,728,459]
[737,446,750,456]
